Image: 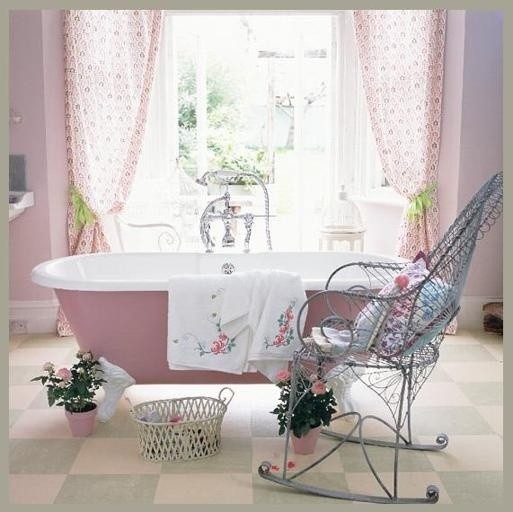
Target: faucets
[199,195,229,254]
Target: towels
[165,269,309,385]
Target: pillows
[355,256,454,357]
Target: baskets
[123,387,234,463]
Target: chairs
[257,169,502,505]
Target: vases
[67,403,98,437]
[287,426,321,456]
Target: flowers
[273,367,338,439]
[30,352,106,411]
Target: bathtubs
[30,249,451,388]
